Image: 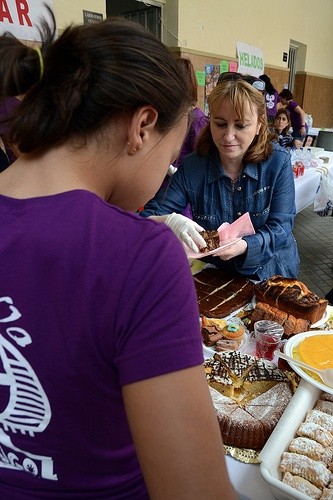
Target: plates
[283,331,333,395]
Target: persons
[259,74,309,149]
[0,17,252,500]
[139,72,299,284]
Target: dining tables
[181,259,333,500]
[288,146,333,215]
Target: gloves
[164,213,207,254]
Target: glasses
[216,72,267,95]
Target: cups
[254,320,285,361]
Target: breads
[193,266,256,350]
[251,274,328,336]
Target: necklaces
[231,176,239,193]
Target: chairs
[316,131,333,152]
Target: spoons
[274,350,333,389]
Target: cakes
[201,351,292,449]
